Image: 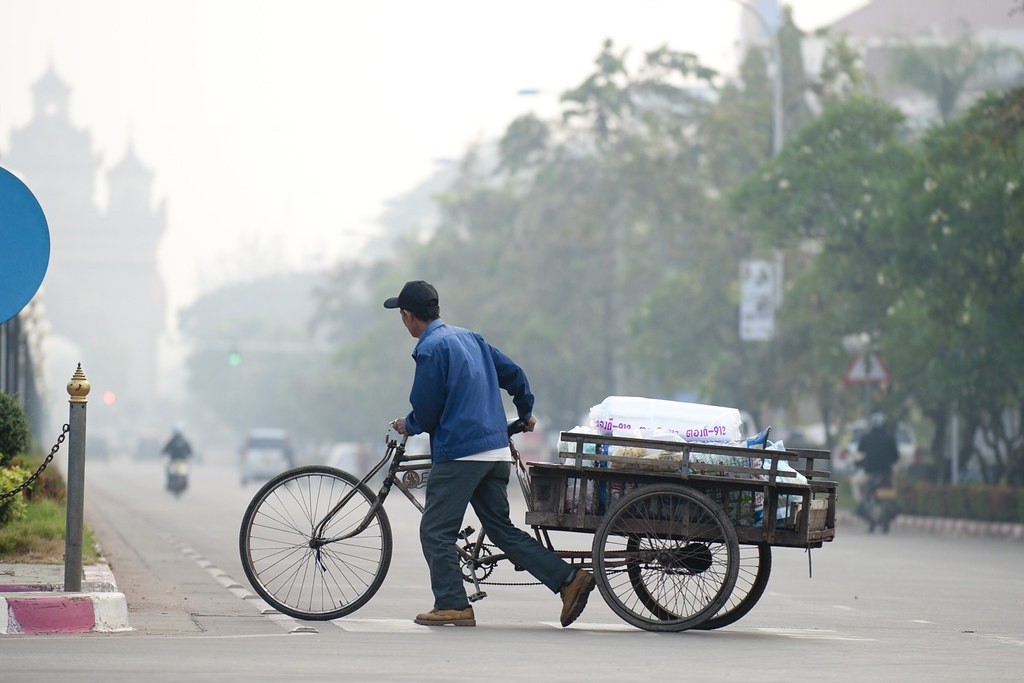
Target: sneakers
[560,569,596,627]
[414,606,476,627]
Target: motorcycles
[860,485,904,535]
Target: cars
[241,431,300,485]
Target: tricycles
[235,424,838,630]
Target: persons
[162,428,195,460]
[850,413,899,513]
[383,279,596,628]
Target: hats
[383,280,440,311]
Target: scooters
[167,456,191,499]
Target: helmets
[869,413,887,432]
